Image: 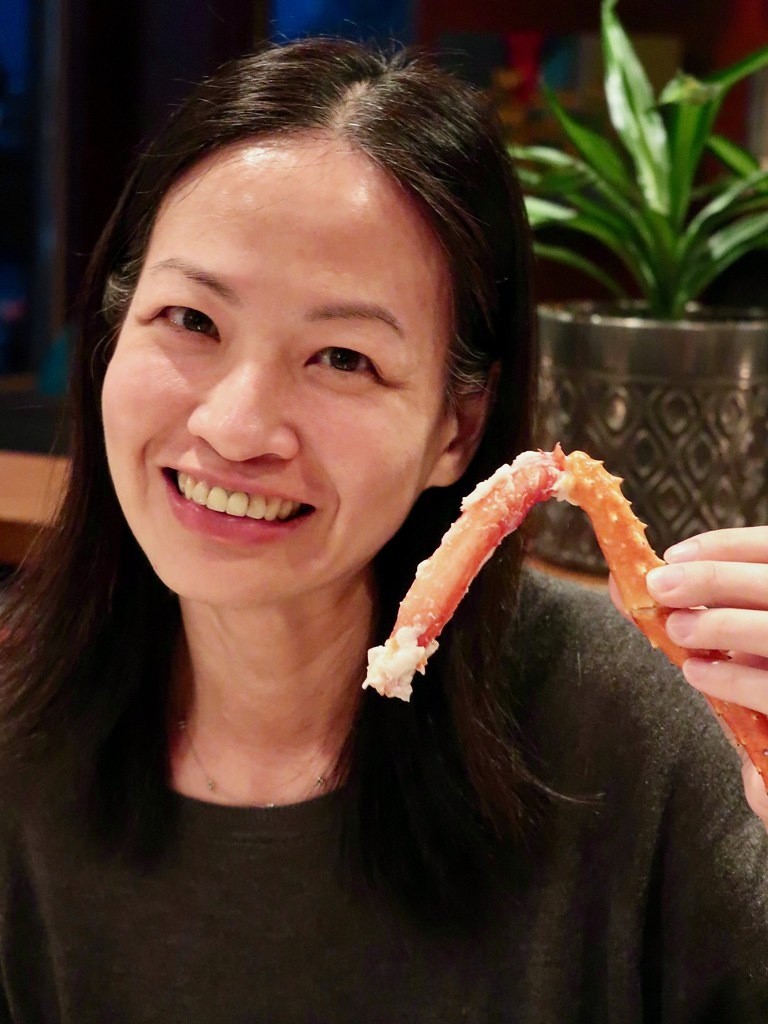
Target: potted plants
[493,0,768,580]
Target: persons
[0,32,767,1024]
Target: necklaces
[175,716,327,807]
